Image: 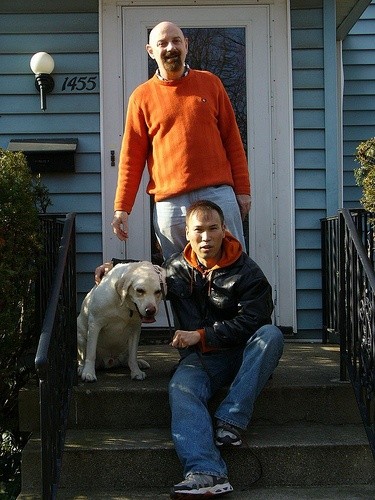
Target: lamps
[29,51,55,113]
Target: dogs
[77,260,169,384]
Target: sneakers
[169,473,234,500]
[216,418,242,447]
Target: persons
[110,20,250,266]
[94,198,285,496]
